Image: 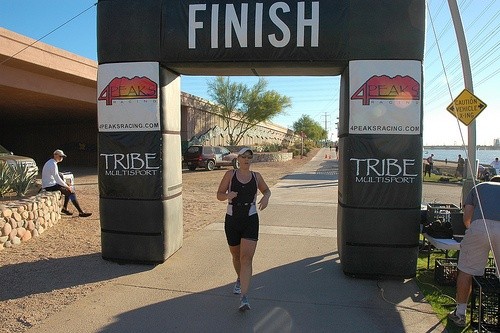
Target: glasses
[241,155,253,159]
[57,156,64,158]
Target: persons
[446,175,500,324]
[490,158,500,175]
[216,148,271,311]
[425,154,434,177]
[455,155,464,179]
[41,149,92,217]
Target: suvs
[184,144,239,170]
[1,146,39,180]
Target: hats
[53,149,67,158]
[237,148,254,156]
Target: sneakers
[448,308,466,327]
[233,280,242,295]
[238,295,251,311]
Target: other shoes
[60,209,73,216]
[79,212,92,218]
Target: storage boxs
[426,203,500,333]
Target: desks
[419,203,461,278]
